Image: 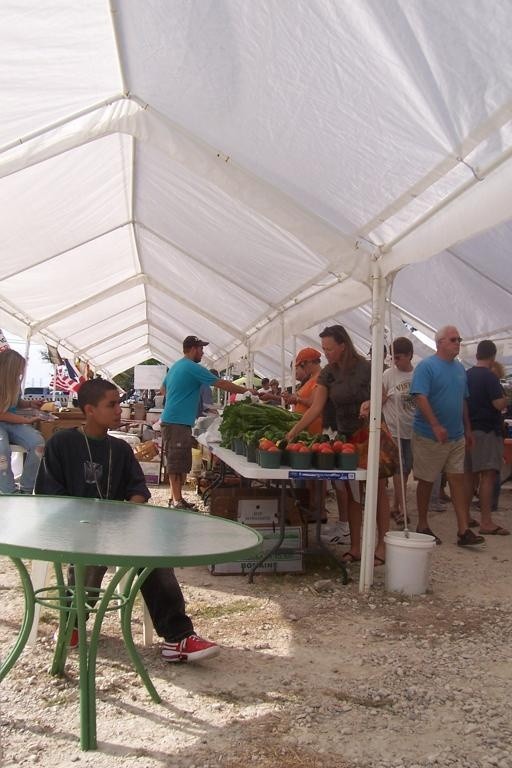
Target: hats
[182,335,210,348]
[293,347,321,365]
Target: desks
[196,434,372,590]
[2,490,265,754]
[496,436,512,513]
[152,416,167,485]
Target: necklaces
[81,426,113,500]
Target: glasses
[451,336,462,343]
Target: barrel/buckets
[383,531,437,597]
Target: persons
[258,326,391,565]
[161,336,260,511]
[383,325,512,546]
[35,378,221,664]
[1,350,46,496]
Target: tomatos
[260,438,357,454]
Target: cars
[22,385,66,398]
[119,394,155,412]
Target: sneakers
[174,499,199,512]
[320,528,351,544]
[456,529,485,546]
[392,510,411,525]
[160,635,220,664]
[168,498,195,508]
[415,526,442,546]
[52,622,80,648]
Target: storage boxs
[197,470,320,578]
[13,398,164,488]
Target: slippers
[480,525,510,537]
[373,553,385,566]
[469,517,480,528]
[342,552,361,562]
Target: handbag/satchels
[355,408,404,477]
